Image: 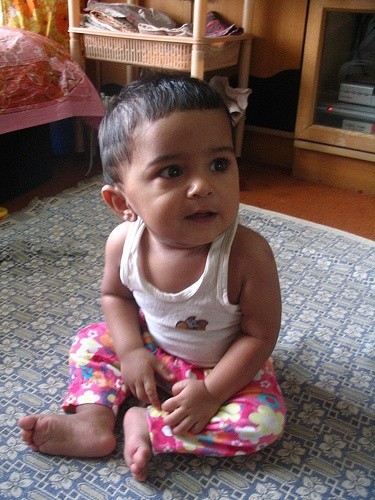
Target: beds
[1,25,105,165]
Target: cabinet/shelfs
[66,0,256,160]
[292,0,375,163]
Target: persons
[17,73,293,482]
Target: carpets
[1,168,375,500]
